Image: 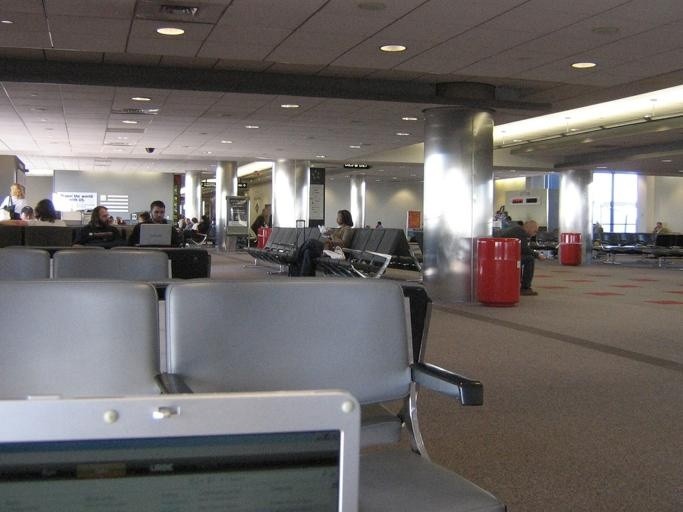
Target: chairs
[586,232,683,271]
[529,231,557,256]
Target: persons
[495,219,546,296]
[128,201,180,248]
[0,183,67,226]
[73,206,122,245]
[652,221,669,242]
[251,208,269,247]
[177,215,215,248]
[365,224,370,228]
[279,210,354,278]
[376,221,383,228]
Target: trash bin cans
[559,232,581,266]
[257,227,272,249]
[476,237,522,307]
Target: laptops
[135,224,173,247]
[0,390,361,512]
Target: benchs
[0,279,505,510]
[2,218,217,281]
[242,225,427,282]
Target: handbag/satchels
[4,204,15,218]
[286,245,299,263]
[322,246,346,260]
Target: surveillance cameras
[145,148,154,153]
